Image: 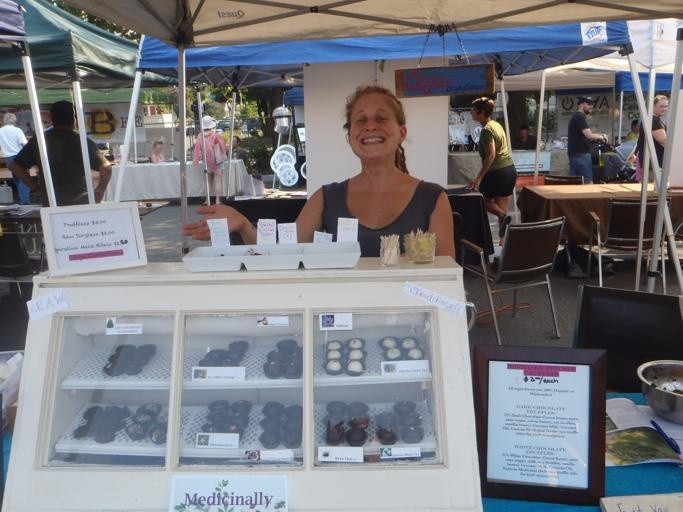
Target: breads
[376,400,423,444]
[104,344,155,376]
[326,338,366,377]
[199,341,249,367]
[74,403,168,444]
[263,340,303,379]
[206,399,251,433]
[259,402,303,448]
[380,336,424,360]
[326,400,369,447]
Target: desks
[448,151,483,187]
[512,148,552,173]
[480,391,683,511]
[516,183,682,246]
[221,187,494,265]
[101,159,248,204]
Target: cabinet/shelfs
[0,253,485,510]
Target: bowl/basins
[636,360,682,423]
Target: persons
[512,124,538,149]
[192,115,227,206]
[181,85,456,261]
[637,95,668,182]
[13,101,112,207]
[567,97,604,183]
[231,135,249,166]
[150,140,165,162]
[466,97,517,247]
[624,120,641,142]
[0,113,31,205]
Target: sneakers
[498,213,511,238]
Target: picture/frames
[473,349,607,506]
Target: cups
[404,233,435,263]
[379,243,400,267]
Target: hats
[203,116,216,130]
[577,96,593,105]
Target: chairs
[575,197,672,296]
[572,284,683,394]
[461,215,567,347]
[543,174,584,185]
